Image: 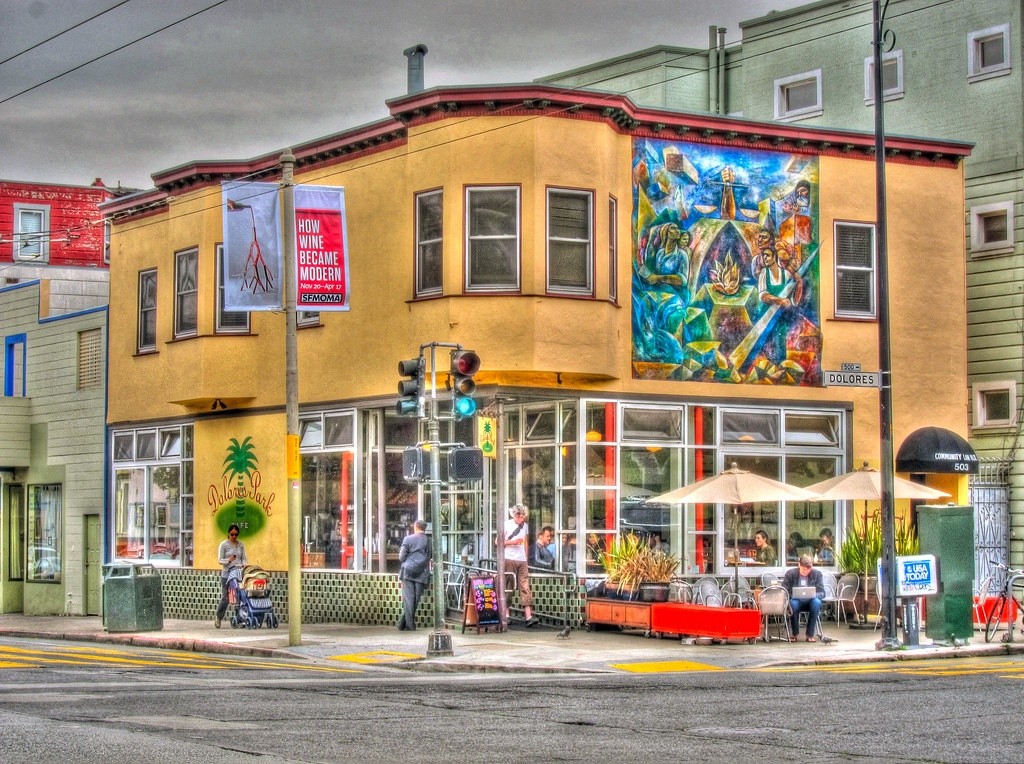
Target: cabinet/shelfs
[582,595,651,637]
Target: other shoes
[788,636,797,642]
[806,635,814,641]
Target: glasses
[228,533,239,536]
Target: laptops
[795,547,813,559]
[792,586,816,599]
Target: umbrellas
[647,466,824,607]
[586,475,660,529]
[803,463,953,629]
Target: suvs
[28,540,192,586]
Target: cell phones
[233,554,236,558]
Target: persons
[751,530,776,567]
[396,521,433,630]
[783,556,826,643]
[589,534,605,563]
[214,525,248,629]
[495,505,540,628]
[817,529,836,566]
[529,526,555,573]
[786,532,815,561]
[547,534,569,572]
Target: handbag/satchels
[400,536,431,578]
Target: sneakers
[526,616,539,627]
[506,617,512,625]
[214,614,221,629]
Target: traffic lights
[452,346,480,419]
[396,354,420,415]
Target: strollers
[226,563,279,629]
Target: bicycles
[984,559,1024,643]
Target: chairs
[673,570,862,643]
